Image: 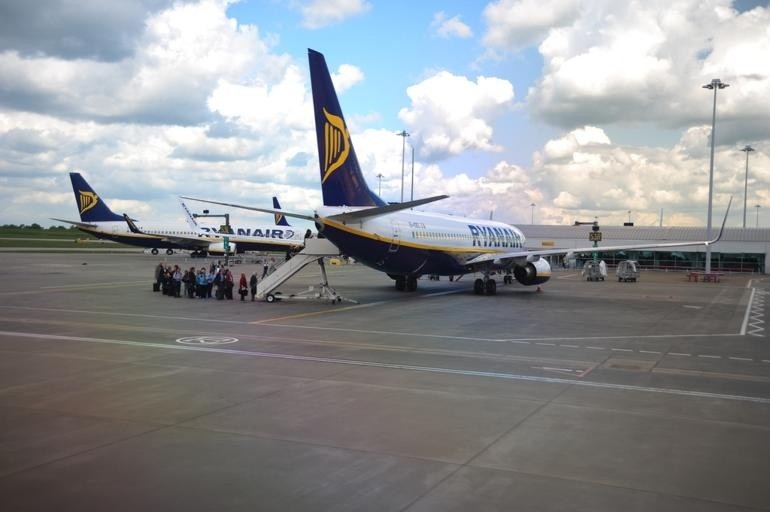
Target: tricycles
[616,260,641,282]
[582,259,608,280]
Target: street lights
[701,77,730,273]
[738,144,755,226]
[376,172,385,197]
[395,129,410,202]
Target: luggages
[216,289,225,299]
[153,283,159,291]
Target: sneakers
[174,295,181,298]
[189,295,211,298]
[240,299,247,303]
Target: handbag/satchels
[239,288,247,296]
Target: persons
[154,254,278,303]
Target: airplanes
[179,46,733,303]
[48,171,328,261]
